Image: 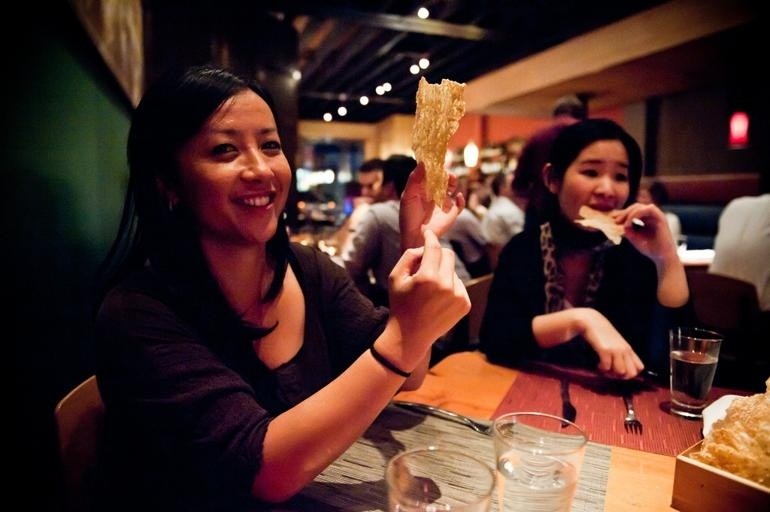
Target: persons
[708,173,770,315]
[95,67,471,508]
[512,96,582,227]
[294,154,524,302]
[479,118,690,382]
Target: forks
[392,400,515,435]
[623,393,643,434]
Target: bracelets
[367,347,414,376]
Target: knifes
[560,373,577,427]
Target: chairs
[53,374,114,512]
[457,273,496,352]
[685,271,770,395]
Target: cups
[494,411,588,512]
[385,447,497,512]
[669,326,721,418]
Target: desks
[279,239,374,278]
